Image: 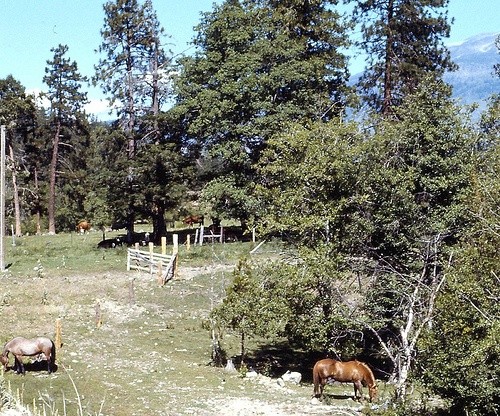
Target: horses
[312,359,380,403]
[0,336,55,375]
